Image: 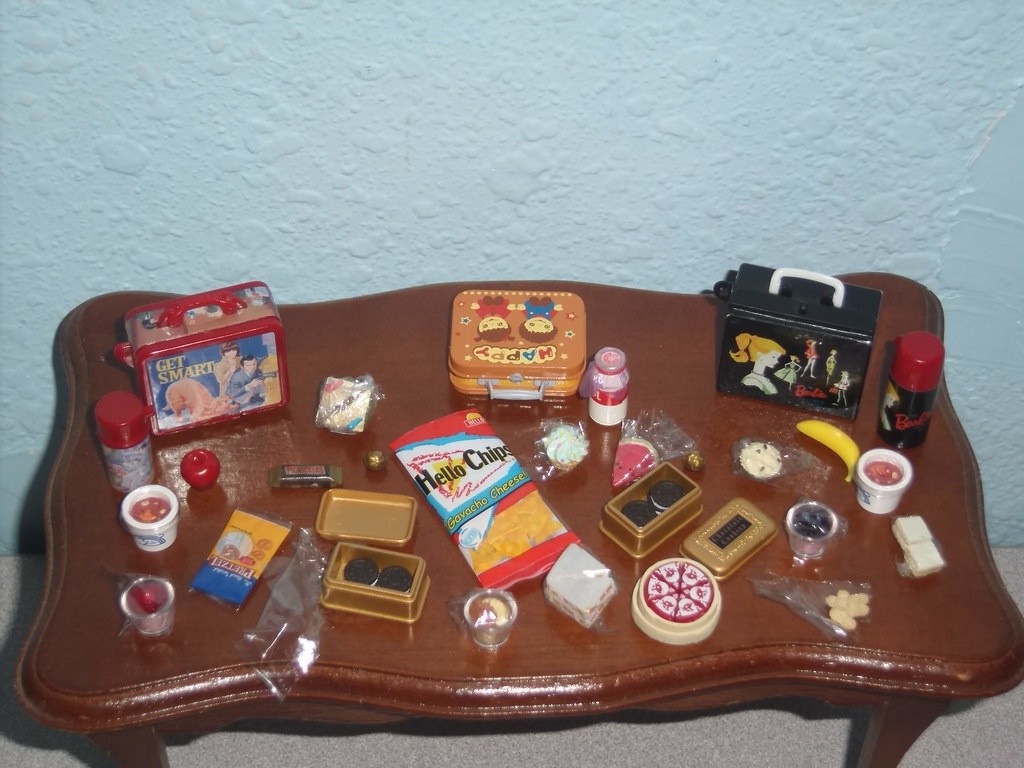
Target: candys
[267,464,342,489]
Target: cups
[785,500,838,559]
[857,448,912,515]
[465,589,516,648]
[122,484,180,552]
[121,578,175,636]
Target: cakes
[631,557,722,645]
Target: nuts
[825,590,871,631]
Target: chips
[471,493,563,572]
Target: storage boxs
[600,462,779,580]
[447,289,586,402]
[114,280,288,437]
[715,263,882,419]
[316,488,431,623]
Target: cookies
[891,515,944,577]
[344,557,414,592]
[621,481,685,529]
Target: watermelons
[611,437,658,488]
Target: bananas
[795,420,860,482]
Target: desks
[16,272,1024,768]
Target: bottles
[96,390,154,495]
[877,330,944,448]
[589,347,629,425]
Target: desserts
[546,543,618,630]
[739,441,783,478]
[542,423,589,473]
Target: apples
[181,448,219,488]
